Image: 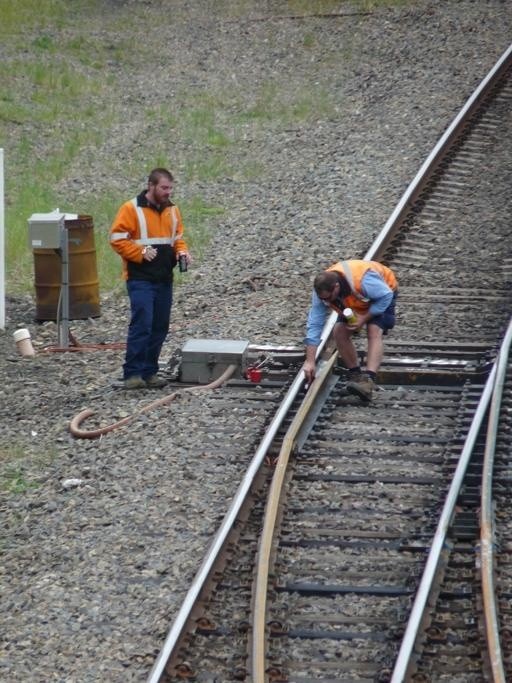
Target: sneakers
[340,370,376,402]
[125,373,168,389]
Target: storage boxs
[182,337,251,384]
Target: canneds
[178,251,187,272]
[343,308,357,324]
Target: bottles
[178,250,188,273]
[342,308,355,326]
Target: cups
[249,369,262,382]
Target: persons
[303,259,400,402]
[107,167,193,389]
